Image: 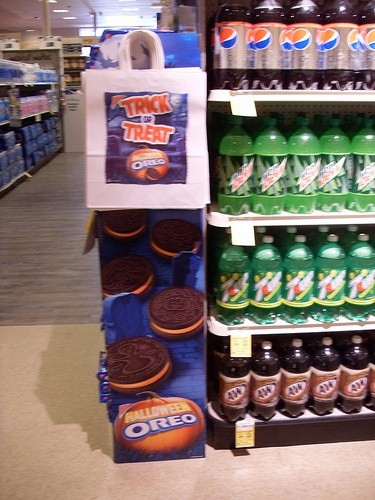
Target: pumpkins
[116,390,205,454]
[127,144,169,181]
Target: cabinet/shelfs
[193,0,375,451]
[0,35,87,195]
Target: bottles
[206,1,374,423]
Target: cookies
[97,210,204,392]
